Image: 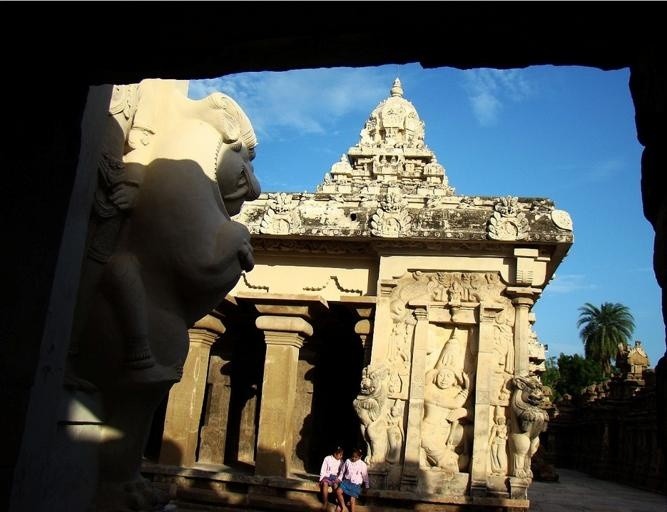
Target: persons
[489,418,511,471]
[385,407,405,464]
[319,446,344,512]
[421,368,476,472]
[336,448,369,512]
